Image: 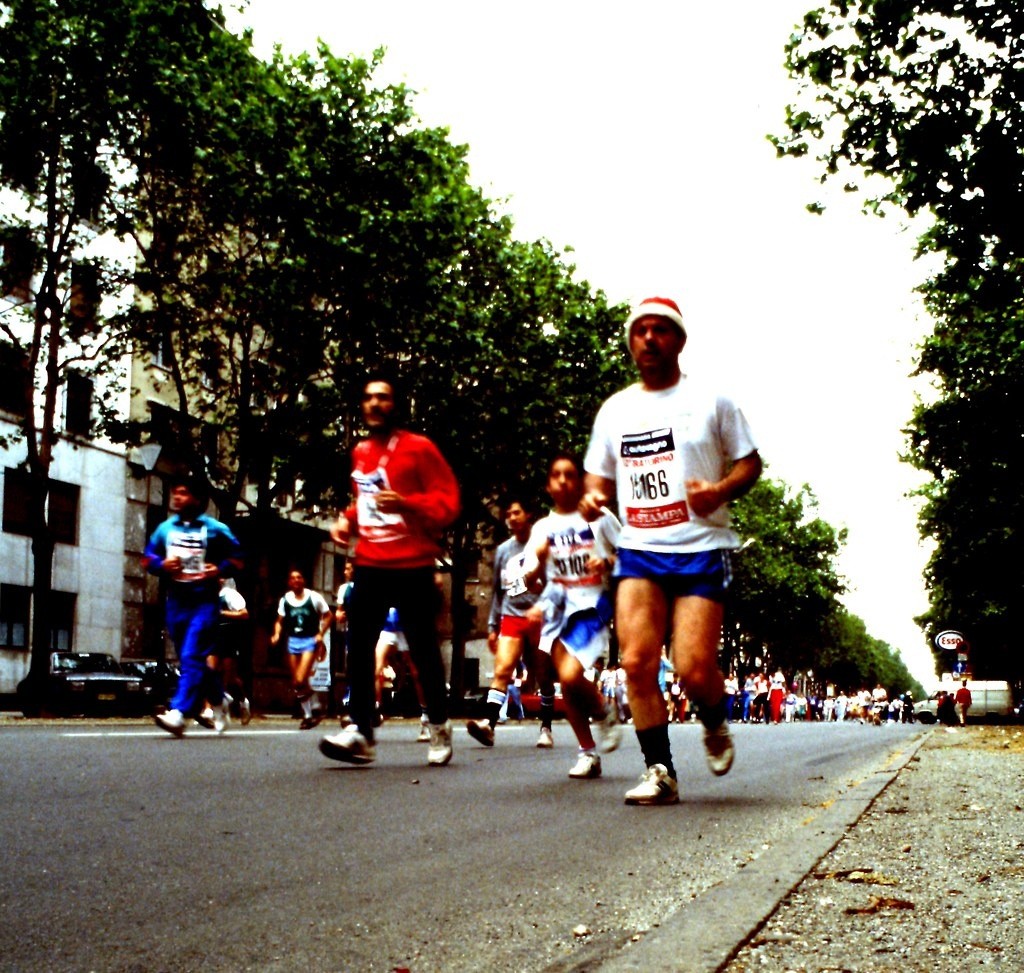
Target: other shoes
[311,703,325,720]
[299,717,314,730]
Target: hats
[624,296,687,355]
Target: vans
[911,681,1012,724]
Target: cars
[17,650,181,718]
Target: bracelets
[604,558,611,570]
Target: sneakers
[318,724,377,765]
[466,718,495,746]
[567,754,602,778]
[416,722,431,743]
[536,727,555,748]
[596,712,623,753]
[427,719,453,766]
[623,764,680,805]
[212,698,229,732]
[155,709,187,738]
[701,718,736,777]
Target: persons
[597,661,807,726]
[139,476,243,734]
[465,457,622,778]
[335,558,430,742]
[194,578,251,726]
[271,570,333,730]
[937,681,972,727]
[579,296,763,804]
[318,374,460,766]
[808,682,914,724]
